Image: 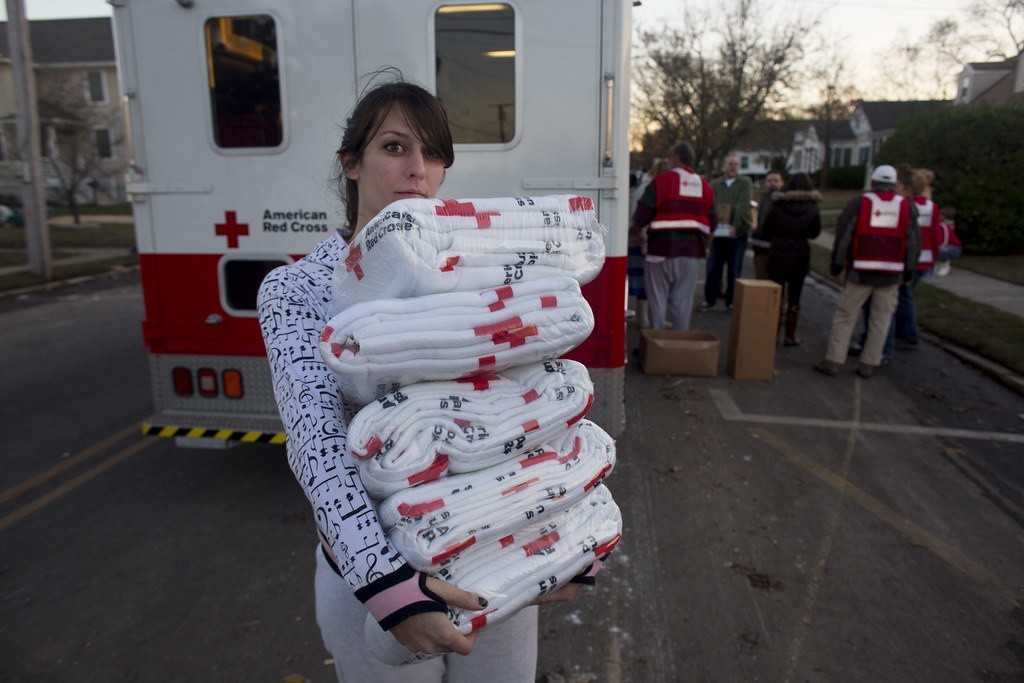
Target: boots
[777,320,782,345]
[784,304,801,345]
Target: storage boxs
[639,329,720,376]
[728,278,782,381]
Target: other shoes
[725,303,734,314]
[858,363,874,377]
[698,301,716,311]
[813,359,839,376]
[633,349,639,356]
[889,339,919,350]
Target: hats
[872,164,897,184]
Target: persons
[255,82,611,683]
[628,140,962,381]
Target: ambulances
[103,0,636,450]
